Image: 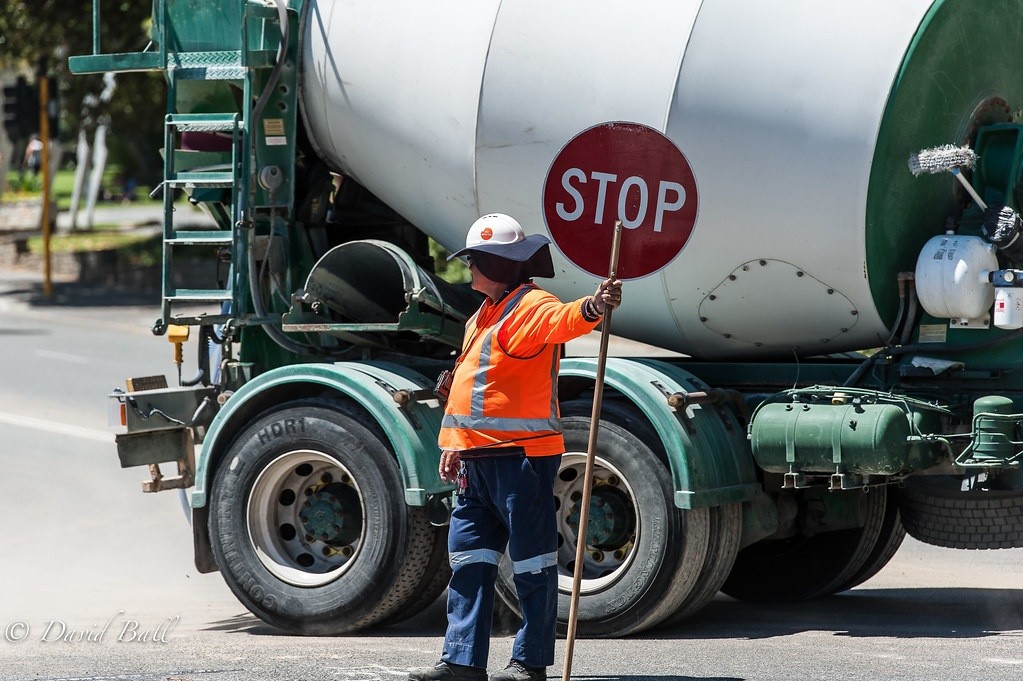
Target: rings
[618,296,620,301]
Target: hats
[445,212,555,278]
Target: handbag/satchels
[432,369,453,402]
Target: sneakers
[490,658,547,681]
[408,659,488,681]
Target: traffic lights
[1,74,44,143]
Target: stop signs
[542,119,707,281]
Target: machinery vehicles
[74,0,1023,679]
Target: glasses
[466,255,477,270]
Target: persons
[407,214,623,681]
[24,134,45,176]
